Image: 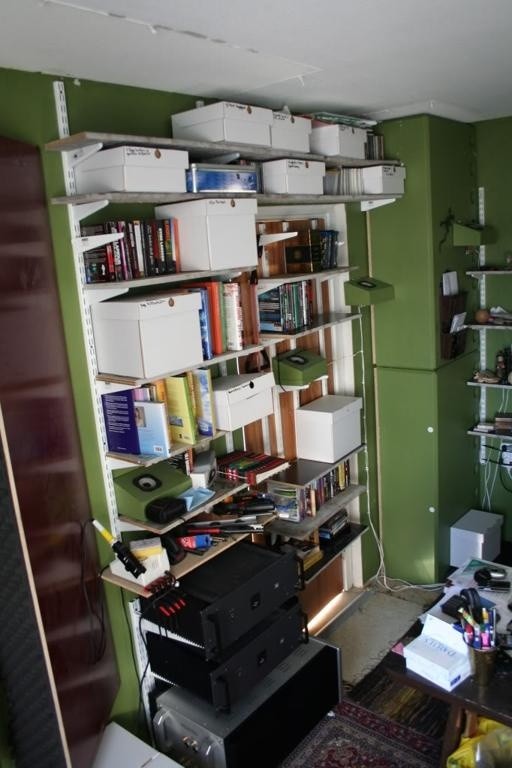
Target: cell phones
[479,579,511,592]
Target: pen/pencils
[452,605,497,650]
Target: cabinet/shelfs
[171,101,273,146]
[270,110,312,154]
[154,198,260,273]
[39,131,403,748]
[310,123,368,160]
[361,164,406,195]
[465,270,512,441]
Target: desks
[382,568,512,768]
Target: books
[217,449,292,485]
[312,229,338,270]
[473,417,512,433]
[81,218,244,458]
[258,278,313,336]
[267,458,350,573]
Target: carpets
[278,698,443,768]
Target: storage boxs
[260,158,326,195]
[186,163,260,193]
[92,720,185,768]
[214,371,276,431]
[293,393,363,464]
[73,145,189,193]
[449,509,504,568]
[90,291,204,380]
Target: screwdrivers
[188,528,264,534]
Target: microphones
[90,518,145,578]
